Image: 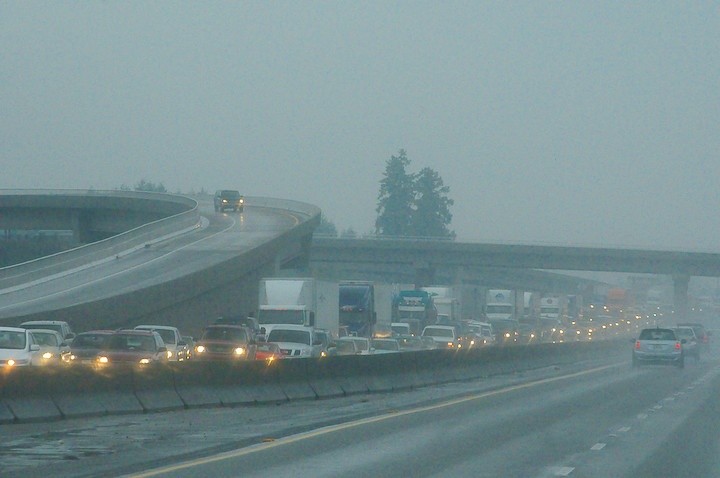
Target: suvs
[213,189,245,213]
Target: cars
[629,326,688,368]
[0,273,615,369]
[662,327,703,362]
[677,322,712,350]
